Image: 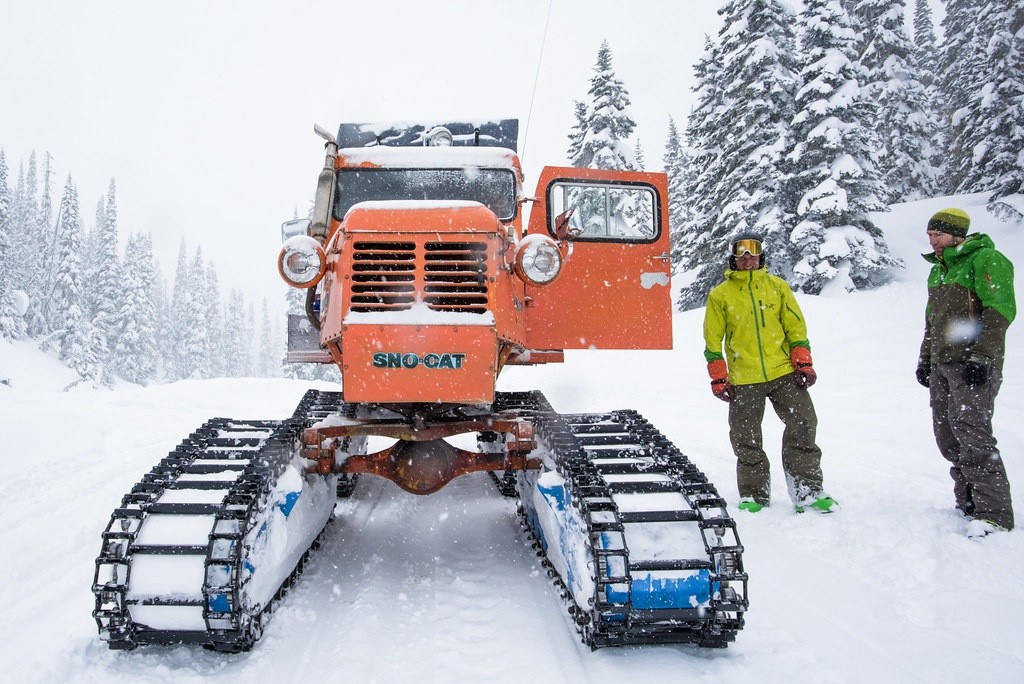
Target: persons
[915,207,1017,545]
[703,231,841,515]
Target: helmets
[733,229,764,245]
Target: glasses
[732,240,763,257]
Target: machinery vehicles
[91,119,749,654]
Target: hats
[927,208,970,238]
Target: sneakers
[794,492,841,514]
[967,518,1011,541]
[738,498,763,512]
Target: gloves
[707,359,735,402]
[790,346,817,391]
[916,367,932,388]
[962,361,988,387]
